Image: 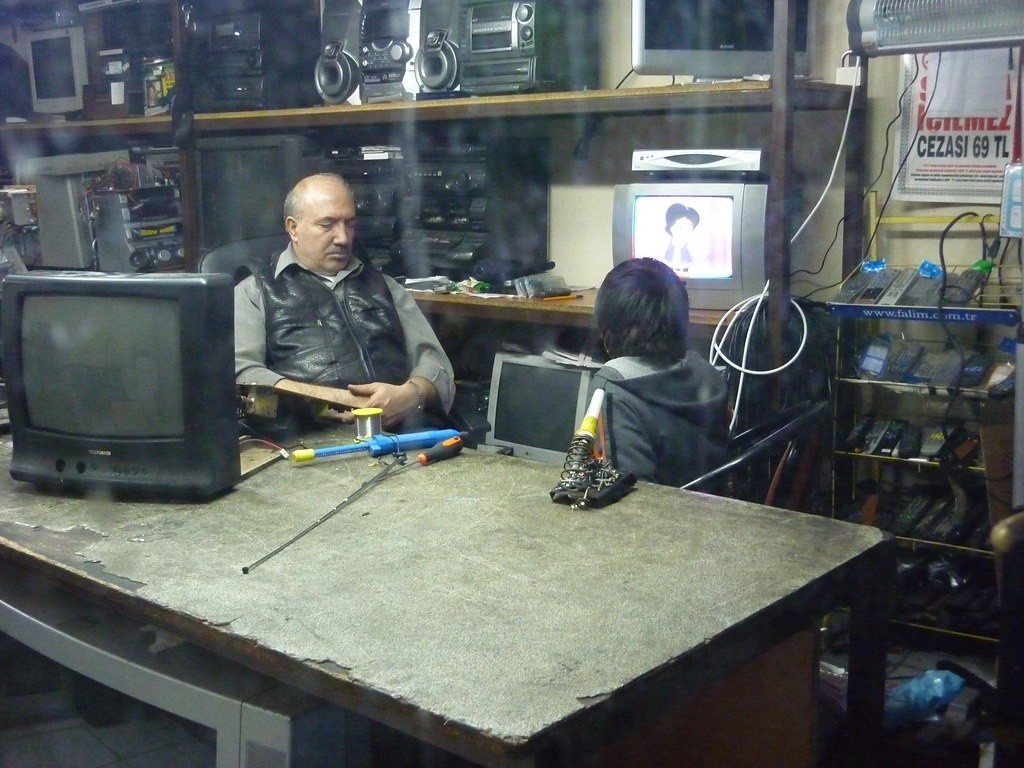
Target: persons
[233,172,456,439]
[584,259,730,496]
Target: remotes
[831,268,1016,589]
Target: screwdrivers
[376,436,464,481]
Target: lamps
[846,0,1024,58]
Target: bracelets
[407,380,424,415]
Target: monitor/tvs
[630,0,813,81]
[613,180,771,311]
[194,135,327,268]
[0,270,245,504]
[486,352,601,465]
[24,26,93,120]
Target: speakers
[314,0,464,104]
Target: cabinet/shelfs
[826,192,1024,694]
[0,0,870,509]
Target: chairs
[677,398,832,509]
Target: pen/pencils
[543,294,584,301]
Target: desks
[0,412,896,768]
